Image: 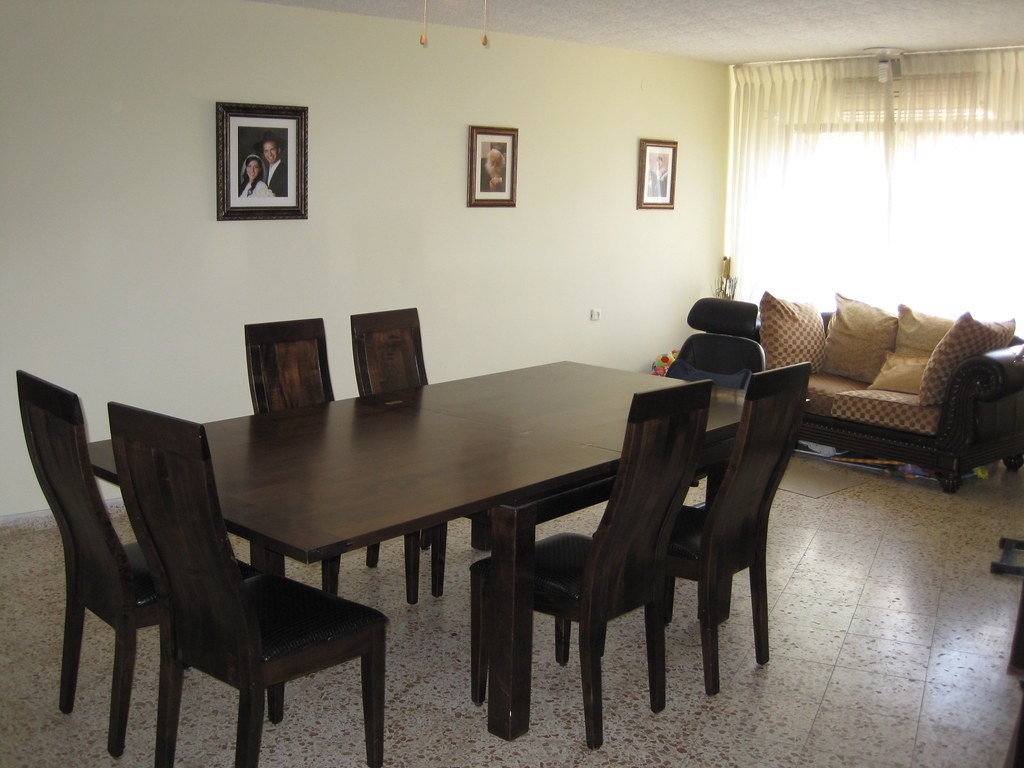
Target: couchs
[754,312,1024,493]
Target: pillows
[761,292,825,375]
[869,303,957,393]
[820,292,898,384]
[918,314,1015,407]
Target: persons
[238,132,288,197]
[646,156,667,197]
[481,146,506,193]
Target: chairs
[108,401,384,768]
[661,297,766,390]
[471,379,713,751]
[351,309,448,596]
[17,369,287,758]
[582,361,809,696]
[243,318,421,604]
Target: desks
[92,361,748,741]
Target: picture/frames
[215,102,309,220]
[467,124,518,207]
[636,138,678,209]
